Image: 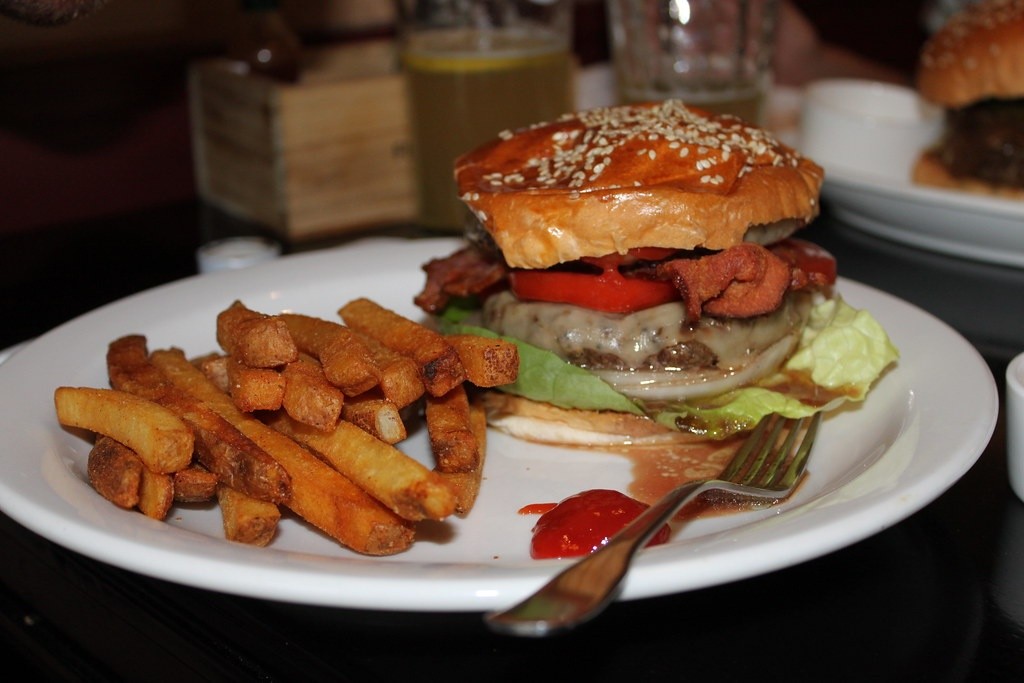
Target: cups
[398,0,577,231]
[606,0,778,128]
[1005,352,1024,502]
[800,78,940,181]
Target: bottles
[186,0,407,244]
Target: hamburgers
[410,97,898,448]
[906,0,1024,201]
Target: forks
[483,412,824,639]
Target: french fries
[53,298,520,557]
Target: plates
[773,126,1024,267]
[576,63,800,109]
[0,237,999,611]
